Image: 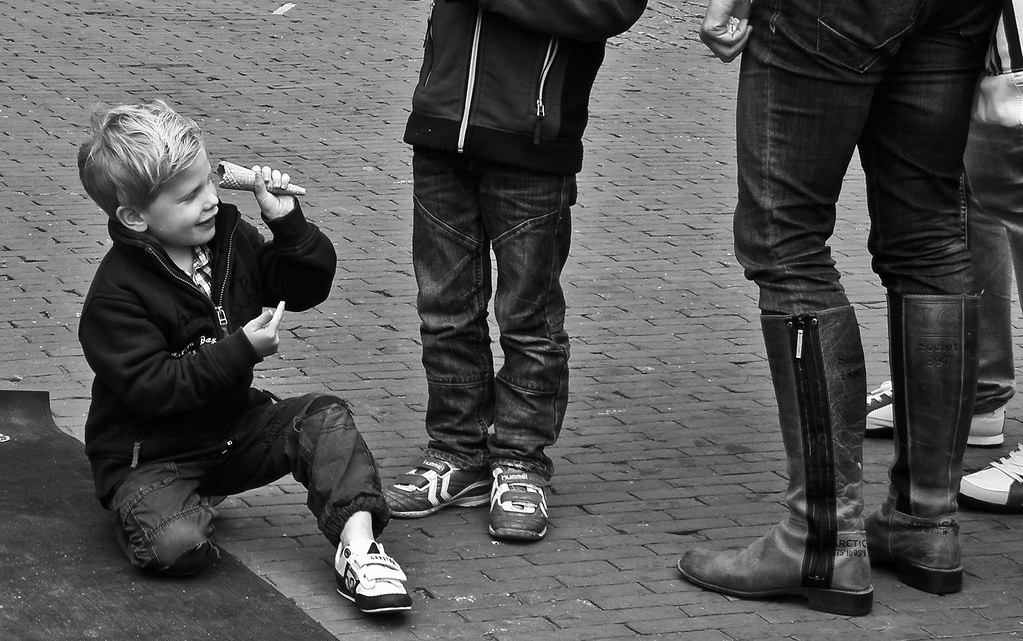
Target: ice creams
[212,160,306,196]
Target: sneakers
[959,435,1023,515]
[489,464,548,541]
[866,375,1005,445]
[334,541,413,615]
[381,455,493,519]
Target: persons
[672,0,991,619]
[864,0,1022,513]
[76,97,415,616]
[379,0,651,541]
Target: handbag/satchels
[962,66,1023,234]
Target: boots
[857,288,982,598]
[679,307,876,613]
